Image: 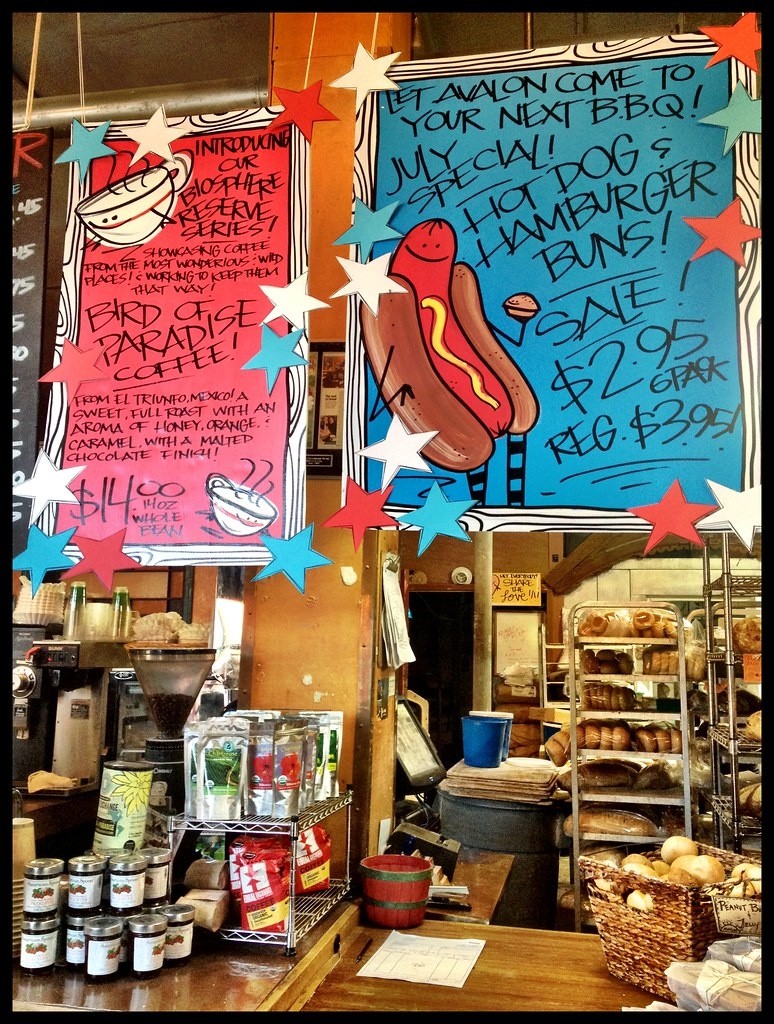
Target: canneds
[20,848,196,985]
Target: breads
[496,609,762,909]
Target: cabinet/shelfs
[165,783,354,957]
[568,600,692,931]
[538,625,581,745]
[687,607,762,852]
[701,534,762,854]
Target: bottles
[63,581,86,640]
[110,586,132,639]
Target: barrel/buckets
[461,711,514,768]
[436,785,559,930]
[361,855,435,929]
